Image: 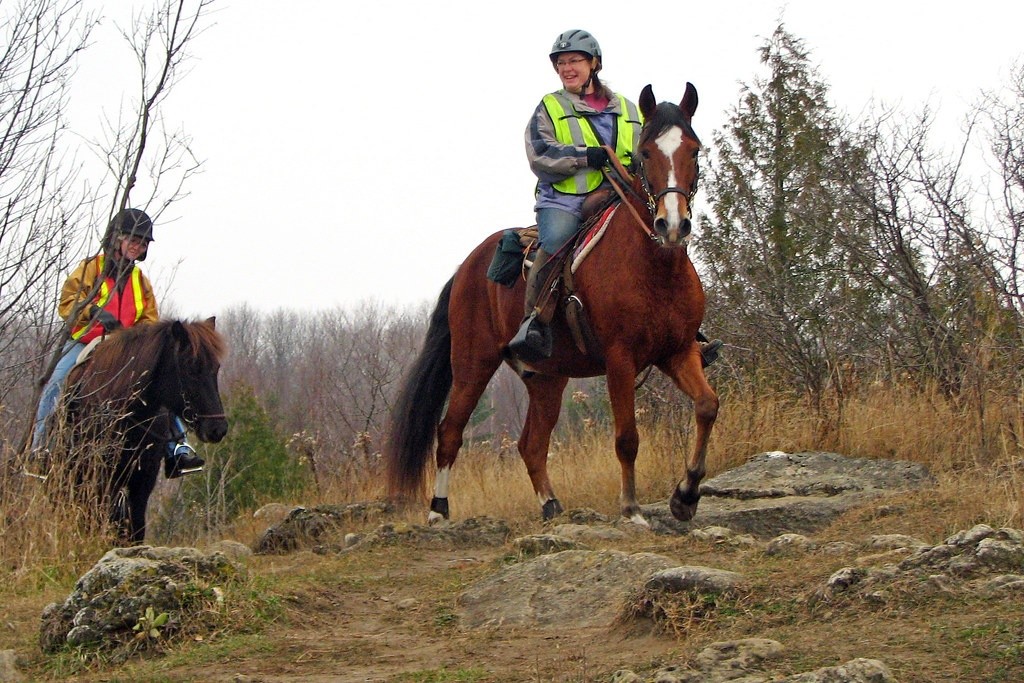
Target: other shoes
[517,323,542,359]
[696,339,722,363]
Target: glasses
[123,235,149,250]
[555,58,591,68]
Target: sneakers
[27,451,48,471]
[165,454,205,479]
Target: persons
[26,207,204,480]
[524,29,722,380]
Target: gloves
[587,147,610,171]
[90,305,118,331]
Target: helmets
[107,209,154,261]
[549,29,601,70]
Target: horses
[48,315,228,551]
[381,82,721,529]
